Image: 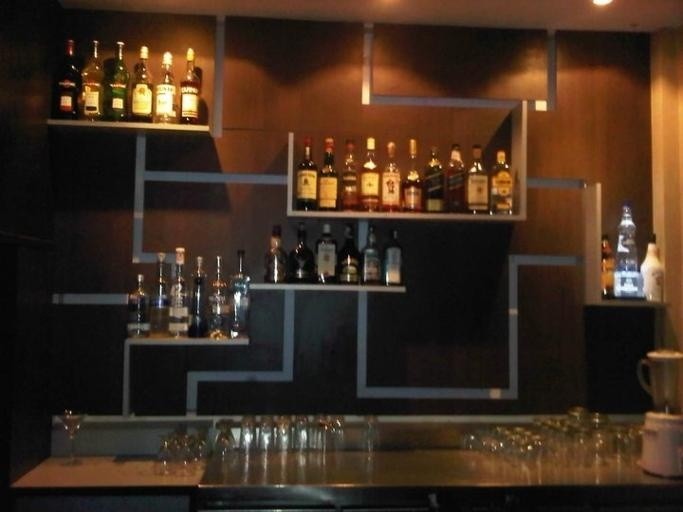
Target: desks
[11,448,680,494]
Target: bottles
[602,203,664,301]
[298,135,514,215]
[266,221,404,287]
[52,36,200,123]
[127,247,249,339]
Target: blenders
[635,347,682,476]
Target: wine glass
[55,409,88,465]
[152,431,207,475]
[211,419,237,462]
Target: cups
[242,414,377,453]
[501,463,634,484]
[237,455,380,480]
[468,405,636,466]
